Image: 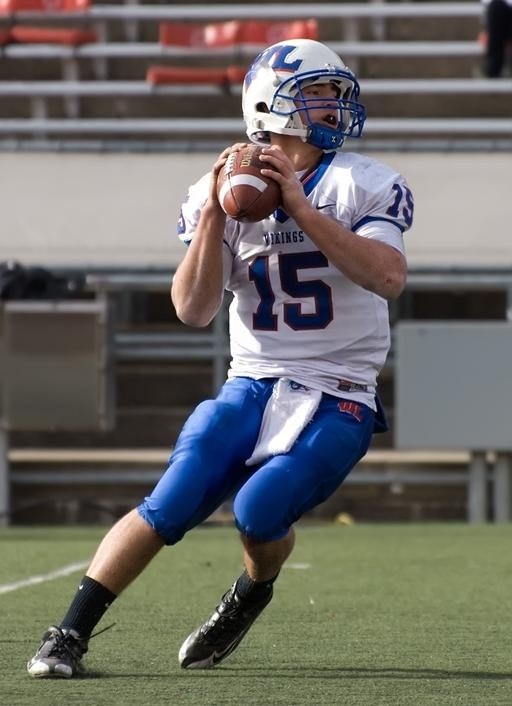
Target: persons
[476,1,512,79]
[25,39,414,678]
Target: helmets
[241,37,366,147]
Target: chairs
[8,0,97,47]
[0,0,10,46]
[227,18,319,85]
[147,19,236,85]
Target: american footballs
[213,139,282,221]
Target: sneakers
[25,626,90,679]
[179,581,276,671]
